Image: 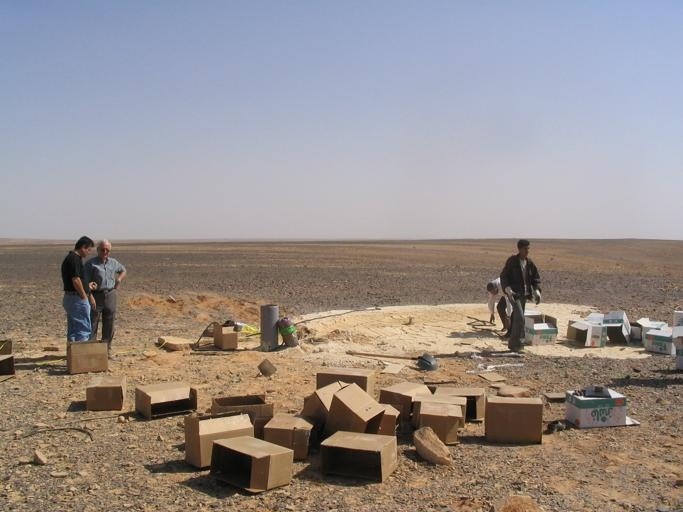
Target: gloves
[504,286,520,306]
[532,288,542,305]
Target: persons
[488,277,513,332]
[60,235,98,344]
[501,239,542,356]
[84,240,127,357]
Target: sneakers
[500,326,527,356]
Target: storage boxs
[0,354,15,382]
[214,326,240,350]
[208,435,294,495]
[264,410,316,459]
[486,396,544,444]
[565,389,641,428]
[184,407,255,470]
[211,391,275,436]
[319,429,402,485]
[134,380,200,420]
[519,307,683,372]
[299,367,485,446]
[0,339,12,354]
[67,341,108,374]
[86,375,128,410]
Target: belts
[93,287,114,294]
